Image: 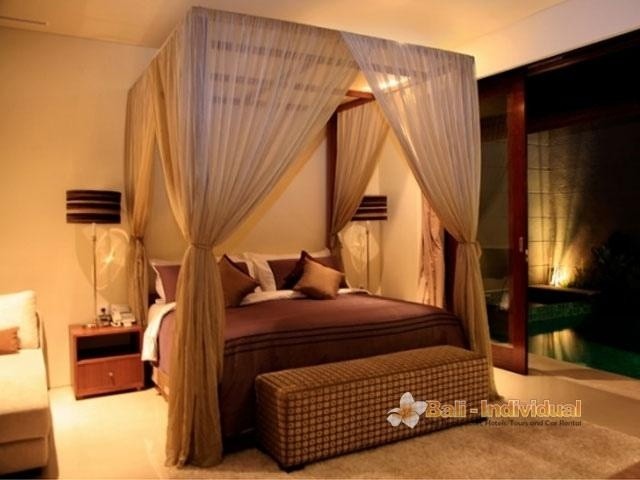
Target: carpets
[145,403,640,479]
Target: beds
[144,287,466,457]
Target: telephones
[111,305,136,324]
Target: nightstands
[69,323,146,400]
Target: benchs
[254,344,490,472]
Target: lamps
[64,190,122,326]
[349,195,387,293]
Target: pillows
[150,247,348,304]
[1,289,39,353]
